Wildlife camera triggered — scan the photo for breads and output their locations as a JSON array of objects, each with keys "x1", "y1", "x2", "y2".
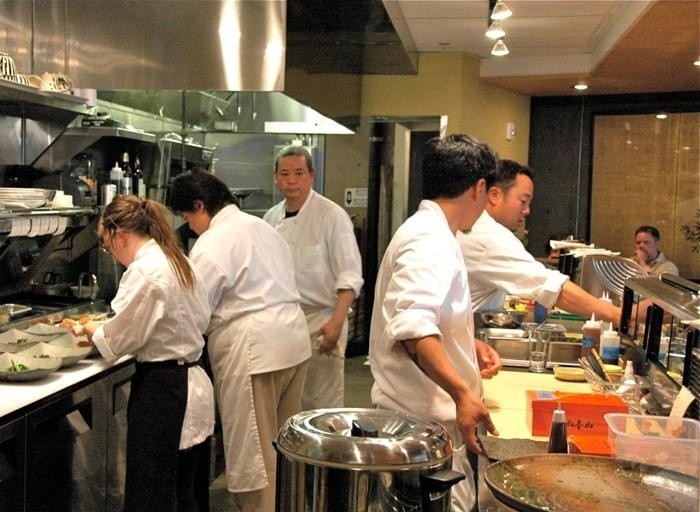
[
  {"x1": 61, "y1": 318, "x2": 76, "y2": 328},
  {"x1": 79, "y1": 313, "x2": 107, "y2": 324}
]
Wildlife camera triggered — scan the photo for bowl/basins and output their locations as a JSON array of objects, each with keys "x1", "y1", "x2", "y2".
[
  {"x1": 1, "y1": 303, "x2": 114, "y2": 381},
  {"x1": 0, "y1": 186, "x2": 55, "y2": 209},
  {"x1": 585, "y1": 372, "x2": 649, "y2": 399},
  {"x1": 70, "y1": 286, "x2": 99, "y2": 300},
  {"x1": 480, "y1": 309, "x2": 528, "y2": 328}
]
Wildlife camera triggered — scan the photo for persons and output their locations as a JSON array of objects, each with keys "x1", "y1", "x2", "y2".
[
  {"x1": 542, "y1": 233, "x2": 574, "y2": 260},
  {"x1": 366, "y1": 131, "x2": 505, "y2": 511},
  {"x1": 260, "y1": 146, "x2": 366, "y2": 410},
  {"x1": 69, "y1": 191, "x2": 217, "y2": 512},
  {"x1": 625, "y1": 225, "x2": 681, "y2": 279},
  {"x1": 450, "y1": 158, "x2": 674, "y2": 330},
  {"x1": 166, "y1": 165, "x2": 315, "y2": 512}
]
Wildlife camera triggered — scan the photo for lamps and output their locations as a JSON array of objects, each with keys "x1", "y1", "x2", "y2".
[{"x1": 483, "y1": 0, "x2": 513, "y2": 59}]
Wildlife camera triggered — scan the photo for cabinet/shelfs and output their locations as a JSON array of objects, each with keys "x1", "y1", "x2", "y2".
[{"x1": 0, "y1": 365, "x2": 136, "y2": 512}]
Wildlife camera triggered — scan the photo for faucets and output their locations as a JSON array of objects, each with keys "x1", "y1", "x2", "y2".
[{"x1": 76, "y1": 269, "x2": 99, "y2": 304}]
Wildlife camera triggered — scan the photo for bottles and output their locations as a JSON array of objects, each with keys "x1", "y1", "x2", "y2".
[
  {"x1": 657, "y1": 332, "x2": 670, "y2": 368},
  {"x1": 624, "y1": 360, "x2": 634, "y2": 376},
  {"x1": 547, "y1": 409, "x2": 569, "y2": 453},
  {"x1": 578, "y1": 311, "x2": 621, "y2": 366},
  {"x1": 82, "y1": 149, "x2": 147, "y2": 207},
  {"x1": 600, "y1": 289, "x2": 612, "y2": 304}
]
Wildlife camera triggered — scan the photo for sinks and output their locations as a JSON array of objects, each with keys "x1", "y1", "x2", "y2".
[
  {"x1": 483, "y1": 330, "x2": 595, "y2": 366},
  {"x1": 1, "y1": 291, "x2": 92, "y2": 309}
]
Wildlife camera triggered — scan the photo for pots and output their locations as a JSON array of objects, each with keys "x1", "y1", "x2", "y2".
[{"x1": 271, "y1": 406, "x2": 465, "y2": 511}]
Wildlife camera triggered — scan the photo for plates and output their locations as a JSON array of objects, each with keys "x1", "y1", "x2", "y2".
[
  {"x1": 227, "y1": 187, "x2": 262, "y2": 194},
  {"x1": 551, "y1": 366, "x2": 586, "y2": 381},
  {"x1": 484, "y1": 453, "x2": 699, "y2": 512}
]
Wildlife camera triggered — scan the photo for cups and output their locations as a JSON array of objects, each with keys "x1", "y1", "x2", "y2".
[
  {"x1": 527, "y1": 327, "x2": 551, "y2": 371},
  {"x1": 0, "y1": 52, "x2": 74, "y2": 95},
  {"x1": 53, "y1": 189, "x2": 74, "y2": 208},
  {"x1": 73, "y1": 87, "x2": 99, "y2": 108}
]
